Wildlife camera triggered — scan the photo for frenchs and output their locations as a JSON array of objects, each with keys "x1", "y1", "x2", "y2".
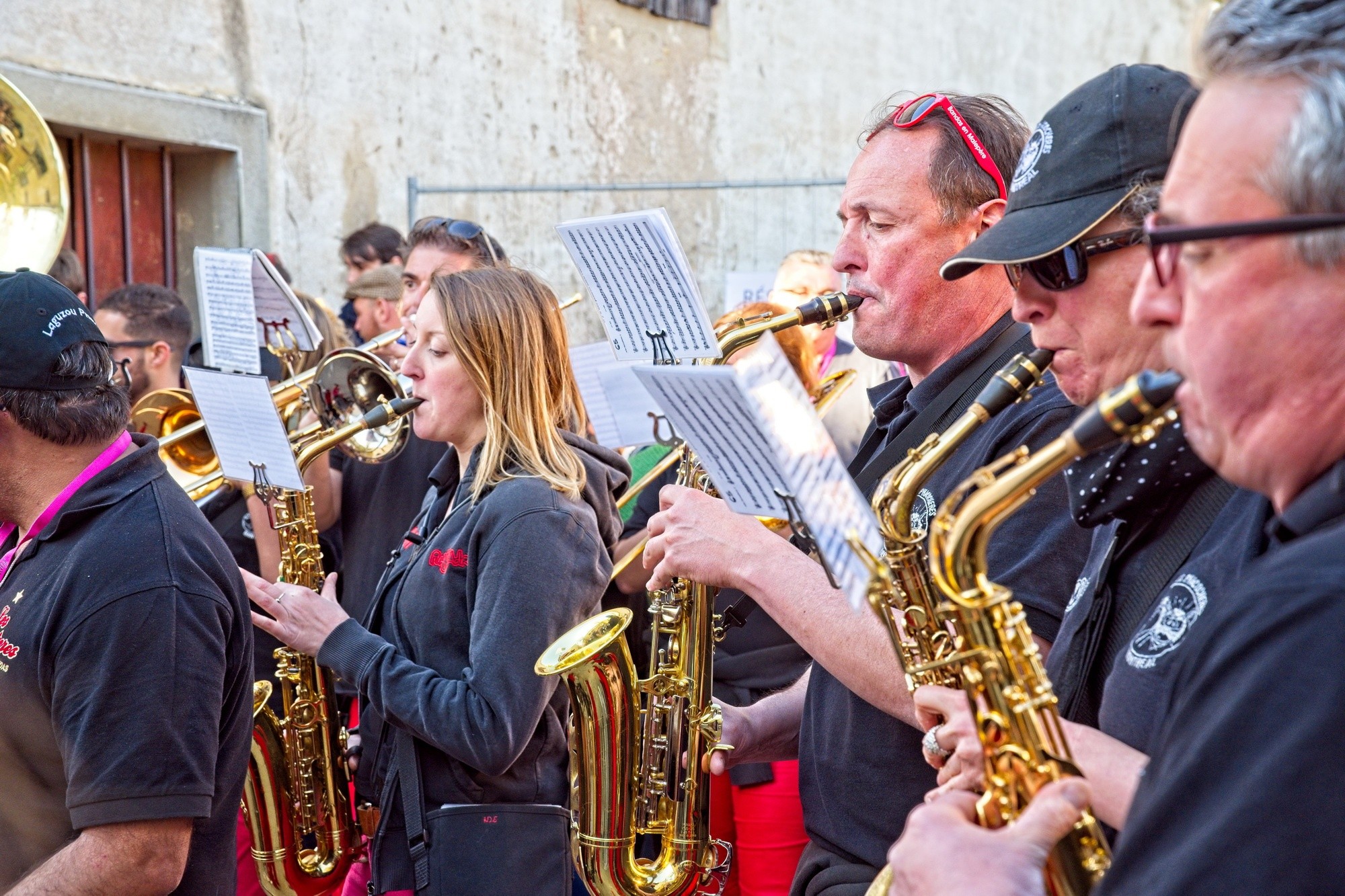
[{"x1": 0, "y1": 72, "x2": 73, "y2": 276}]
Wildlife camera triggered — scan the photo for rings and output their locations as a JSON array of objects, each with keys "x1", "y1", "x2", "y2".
[
  {"x1": 925, "y1": 725, "x2": 952, "y2": 759},
  {"x1": 279, "y1": 592, "x2": 287, "y2": 605}
]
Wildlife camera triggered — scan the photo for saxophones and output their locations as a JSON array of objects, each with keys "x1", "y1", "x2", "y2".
[
  {"x1": 830, "y1": 340, "x2": 1188, "y2": 896},
  {"x1": 237, "y1": 392, "x2": 424, "y2": 896},
  {"x1": 526, "y1": 291, "x2": 868, "y2": 896}
]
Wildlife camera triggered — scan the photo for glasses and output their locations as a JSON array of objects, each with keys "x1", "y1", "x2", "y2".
[
  {"x1": 866, "y1": 93, "x2": 1008, "y2": 202},
  {"x1": 1003, "y1": 227, "x2": 1144, "y2": 290},
  {"x1": 1143, "y1": 212, "x2": 1344, "y2": 288},
  {"x1": 412, "y1": 215, "x2": 500, "y2": 269}
]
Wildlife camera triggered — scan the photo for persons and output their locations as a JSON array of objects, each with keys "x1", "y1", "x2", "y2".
[
  {"x1": 0, "y1": 268, "x2": 257, "y2": 896},
  {"x1": 607, "y1": 2, "x2": 1344, "y2": 896},
  {"x1": 16, "y1": 215, "x2": 634, "y2": 896}
]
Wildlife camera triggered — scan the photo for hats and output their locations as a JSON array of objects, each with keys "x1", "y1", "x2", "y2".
[
  {"x1": 0, "y1": 267, "x2": 118, "y2": 391},
  {"x1": 345, "y1": 266, "x2": 405, "y2": 301},
  {"x1": 939, "y1": 63, "x2": 1203, "y2": 281}
]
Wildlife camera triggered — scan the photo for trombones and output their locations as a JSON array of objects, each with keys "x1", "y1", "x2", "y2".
[
  {"x1": 610, "y1": 367, "x2": 856, "y2": 583},
  {"x1": 126, "y1": 288, "x2": 585, "y2": 512}
]
